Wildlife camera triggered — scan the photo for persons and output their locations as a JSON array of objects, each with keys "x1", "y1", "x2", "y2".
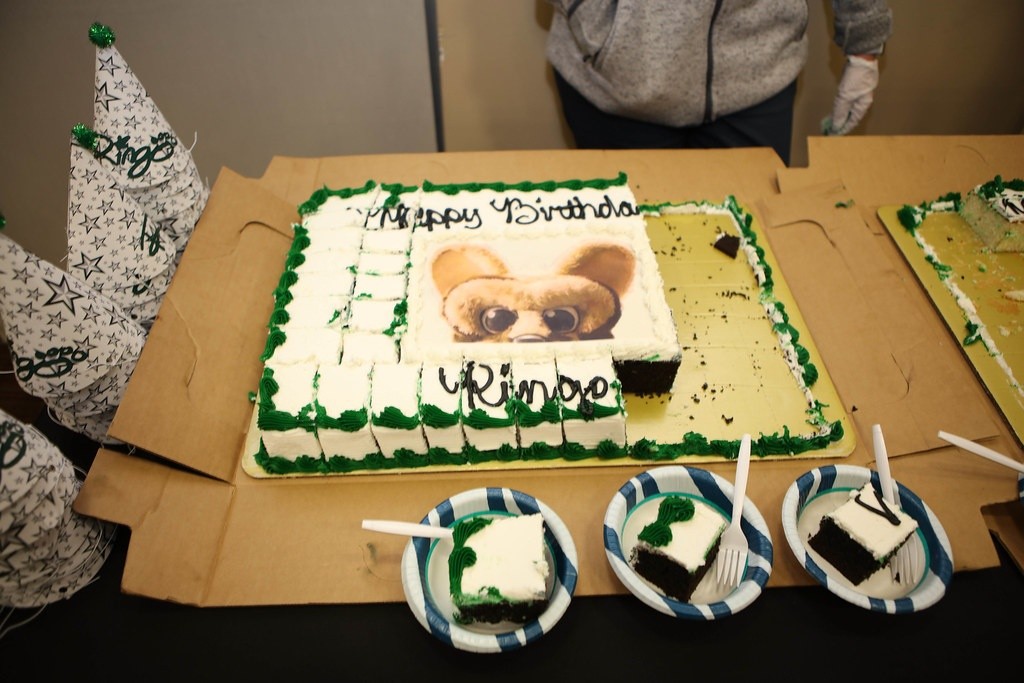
[{"x1": 544, "y1": 0, "x2": 892, "y2": 162}]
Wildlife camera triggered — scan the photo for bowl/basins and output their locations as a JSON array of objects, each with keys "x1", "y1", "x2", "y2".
[
  {"x1": 399, "y1": 484, "x2": 580, "y2": 655},
  {"x1": 780, "y1": 463, "x2": 954, "y2": 616},
  {"x1": 603, "y1": 464, "x2": 773, "y2": 623}
]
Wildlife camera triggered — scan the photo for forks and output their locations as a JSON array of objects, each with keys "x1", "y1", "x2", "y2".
[
  {"x1": 871, "y1": 422, "x2": 919, "y2": 587},
  {"x1": 716, "y1": 432, "x2": 751, "y2": 589}
]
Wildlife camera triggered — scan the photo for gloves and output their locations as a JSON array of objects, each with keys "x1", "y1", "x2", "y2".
[{"x1": 821, "y1": 55, "x2": 878, "y2": 137}]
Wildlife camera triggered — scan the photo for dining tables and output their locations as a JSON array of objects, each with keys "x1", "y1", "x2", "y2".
[{"x1": 0, "y1": 341, "x2": 1024, "y2": 683}]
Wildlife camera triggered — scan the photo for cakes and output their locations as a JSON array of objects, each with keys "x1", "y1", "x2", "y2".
[
  {"x1": 446, "y1": 512, "x2": 548, "y2": 625},
  {"x1": 895, "y1": 173, "x2": 1023, "y2": 395},
  {"x1": 252, "y1": 172, "x2": 842, "y2": 474},
  {"x1": 808, "y1": 483, "x2": 918, "y2": 587},
  {"x1": 627, "y1": 494, "x2": 726, "y2": 605}
]
